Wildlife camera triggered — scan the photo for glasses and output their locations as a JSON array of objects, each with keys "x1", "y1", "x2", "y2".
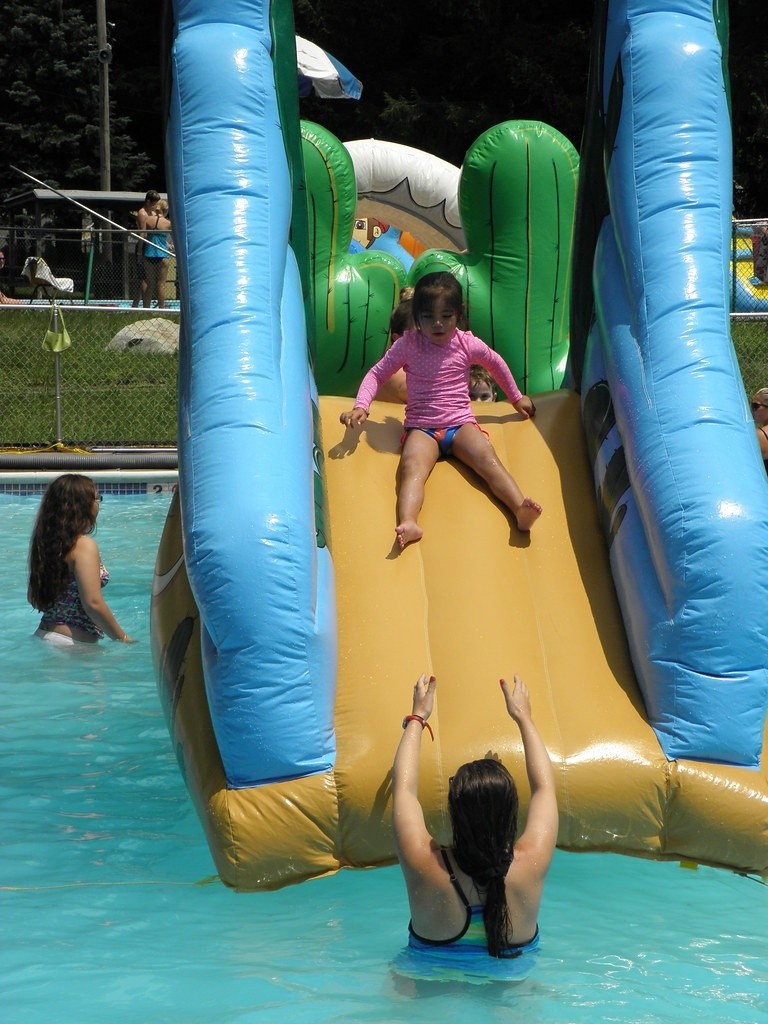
[
  {"x1": 750, "y1": 403, "x2": 768, "y2": 411},
  {"x1": 94, "y1": 494, "x2": 102, "y2": 505}
]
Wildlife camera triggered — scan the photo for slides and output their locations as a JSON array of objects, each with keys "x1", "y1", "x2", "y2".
[{"x1": 145, "y1": 0, "x2": 767, "y2": 894}]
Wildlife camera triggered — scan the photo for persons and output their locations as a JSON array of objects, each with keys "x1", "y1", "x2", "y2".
[
  {"x1": 339, "y1": 272, "x2": 543, "y2": 549},
  {"x1": 132, "y1": 191, "x2": 171, "y2": 309},
  {"x1": 752, "y1": 388, "x2": 768, "y2": 475},
  {"x1": 390, "y1": 671, "x2": 558, "y2": 955},
  {"x1": 27, "y1": 474, "x2": 132, "y2": 649},
  {"x1": 0, "y1": 251, "x2": 25, "y2": 305}
]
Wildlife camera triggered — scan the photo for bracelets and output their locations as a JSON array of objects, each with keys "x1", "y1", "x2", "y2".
[
  {"x1": 115, "y1": 635, "x2": 127, "y2": 642},
  {"x1": 413, "y1": 715, "x2": 424, "y2": 721}
]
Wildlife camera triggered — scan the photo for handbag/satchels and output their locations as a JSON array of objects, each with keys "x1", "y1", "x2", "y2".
[{"x1": 42, "y1": 303, "x2": 71, "y2": 352}]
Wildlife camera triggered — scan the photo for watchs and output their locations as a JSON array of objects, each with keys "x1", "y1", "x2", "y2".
[{"x1": 401, "y1": 716, "x2": 434, "y2": 741}]
